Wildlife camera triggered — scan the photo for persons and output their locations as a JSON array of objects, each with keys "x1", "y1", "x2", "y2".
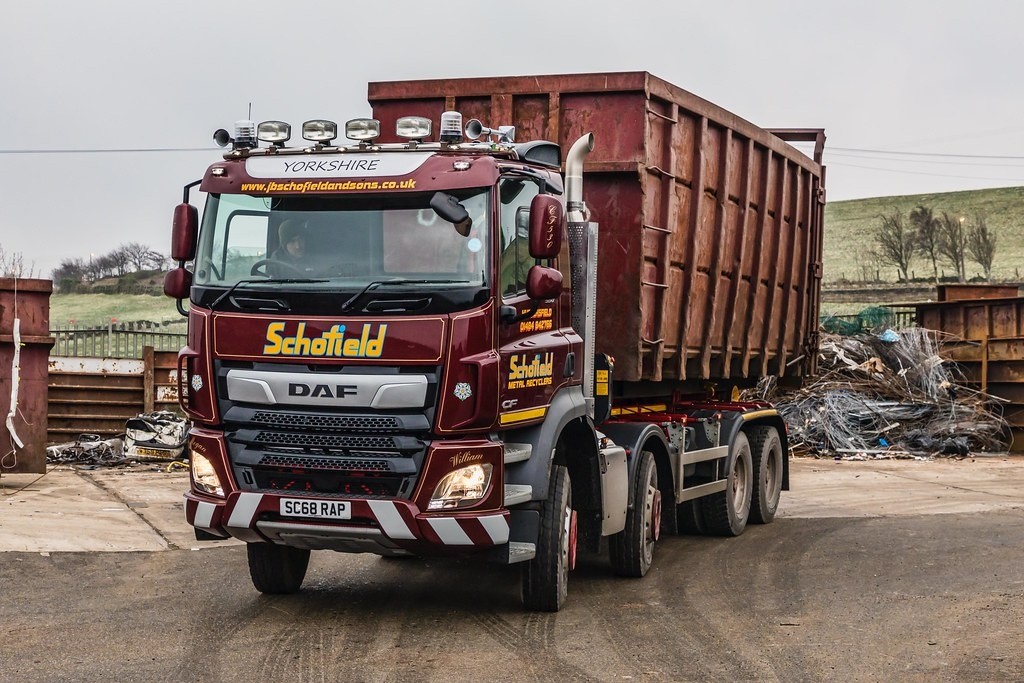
[{"x1": 265, "y1": 219, "x2": 324, "y2": 279}]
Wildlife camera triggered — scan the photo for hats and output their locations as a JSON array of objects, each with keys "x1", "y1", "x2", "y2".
[{"x1": 278, "y1": 219, "x2": 306, "y2": 248}]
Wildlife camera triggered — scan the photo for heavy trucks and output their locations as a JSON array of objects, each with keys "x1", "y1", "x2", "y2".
[{"x1": 164, "y1": 65, "x2": 828, "y2": 613}]
